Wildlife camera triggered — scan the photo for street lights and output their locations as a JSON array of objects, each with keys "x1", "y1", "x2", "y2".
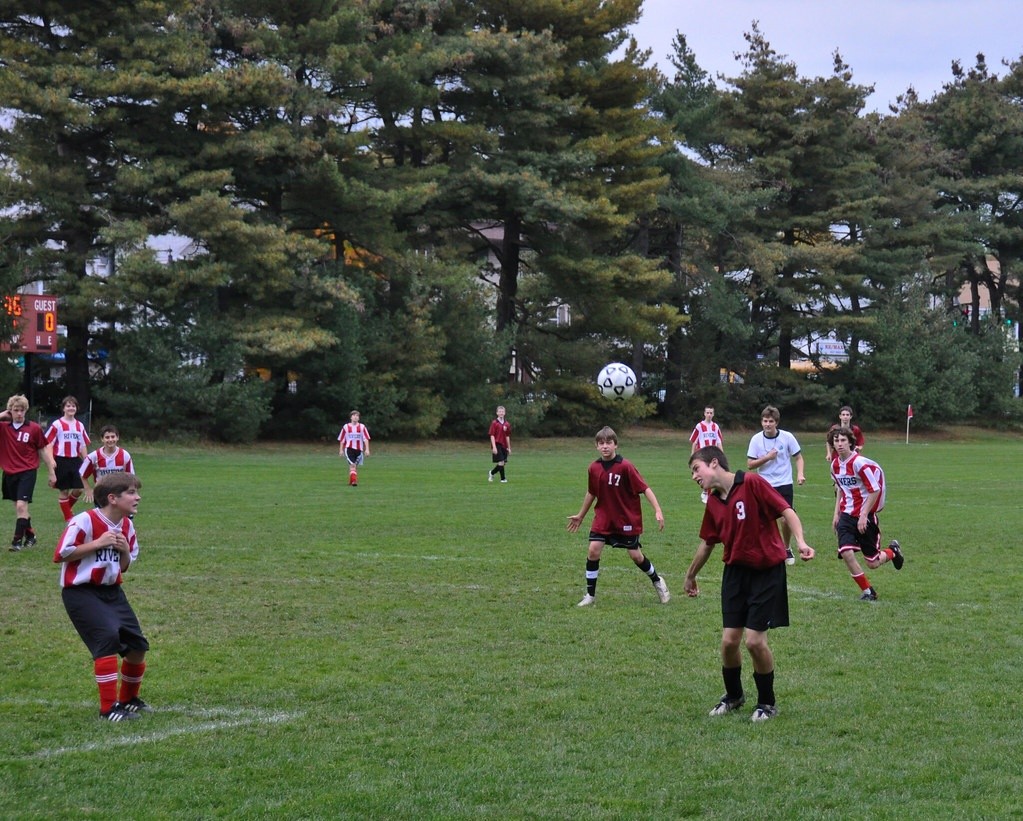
[{"x1": 117, "y1": 240, "x2": 177, "y2": 328}]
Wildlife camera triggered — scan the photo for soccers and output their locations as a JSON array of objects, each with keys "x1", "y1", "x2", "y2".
[{"x1": 597, "y1": 362, "x2": 636, "y2": 402}]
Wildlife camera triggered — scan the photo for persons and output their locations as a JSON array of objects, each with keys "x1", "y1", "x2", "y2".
[
  {"x1": 746, "y1": 405, "x2": 807, "y2": 566},
  {"x1": 827, "y1": 427, "x2": 904, "y2": 602},
  {"x1": 336, "y1": 410, "x2": 371, "y2": 487},
  {"x1": 51, "y1": 472, "x2": 158, "y2": 722},
  {"x1": 0, "y1": 394, "x2": 57, "y2": 552},
  {"x1": 825, "y1": 405, "x2": 865, "y2": 498},
  {"x1": 44, "y1": 395, "x2": 92, "y2": 522},
  {"x1": 566, "y1": 426, "x2": 671, "y2": 607},
  {"x1": 689, "y1": 402, "x2": 725, "y2": 504},
  {"x1": 488, "y1": 405, "x2": 512, "y2": 483},
  {"x1": 79, "y1": 424, "x2": 137, "y2": 525},
  {"x1": 683, "y1": 445, "x2": 815, "y2": 724}
]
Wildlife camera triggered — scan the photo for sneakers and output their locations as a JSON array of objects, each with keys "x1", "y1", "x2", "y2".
[
  {"x1": 653, "y1": 576, "x2": 671, "y2": 604},
  {"x1": 785, "y1": 547, "x2": 795, "y2": 566},
  {"x1": 99, "y1": 697, "x2": 153, "y2": 722},
  {"x1": 888, "y1": 540, "x2": 904, "y2": 570},
  {"x1": 701, "y1": 491, "x2": 708, "y2": 504},
  {"x1": 860, "y1": 586, "x2": 878, "y2": 601},
  {"x1": 709, "y1": 693, "x2": 746, "y2": 716},
  {"x1": 24, "y1": 535, "x2": 37, "y2": 548},
  {"x1": 500, "y1": 479, "x2": 507, "y2": 483},
  {"x1": 488, "y1": 470, "x2": 494, "y2": 482},
  {"x1": 578, "y1": 593, "x2": 595, "y2": 607},
  {"x1": 751, "y1": 703, "x2": 776, "y2": 722},
  {"x1": 9, "y1": 537, "x2": 22, "y2": 552}
]
